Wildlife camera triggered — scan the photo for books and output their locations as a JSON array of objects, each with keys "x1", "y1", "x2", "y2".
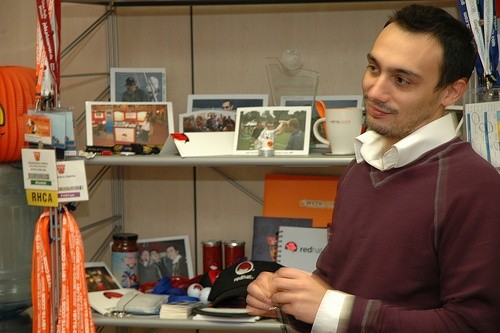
[{"x1": 251, "y1": 171, "x2": 342, "y2": 273}]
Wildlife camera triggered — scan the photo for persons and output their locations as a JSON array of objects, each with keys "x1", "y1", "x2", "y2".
[
  {"x1": 98, "y1": 77, "x2": 304, "y2": 152},
  {"x1": 243, "y1": 4, "x2": 500, "y2": 333},
  {"x1": 137, "y1": 246, "x2": 190, "y2": 284}
]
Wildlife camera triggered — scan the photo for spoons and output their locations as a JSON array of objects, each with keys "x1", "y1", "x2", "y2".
[{"x1": 316, "y1": 100, "x2": 327, "y2": 138}]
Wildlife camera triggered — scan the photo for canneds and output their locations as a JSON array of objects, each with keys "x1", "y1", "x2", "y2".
[{"x1": 111, "y1": 232, "x2": 140, "y2": 289}]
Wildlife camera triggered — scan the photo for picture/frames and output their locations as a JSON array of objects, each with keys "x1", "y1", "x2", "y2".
[
  {"x1": 187, "y1": 92, "x2": 269, "y2": 142},
  {"x1": 108, "y1": 234, "x2": 195, "y2": 285},
  {"x1": 85, "y1": 100, "x2": 175, "y2": 154},
  {"x1": 279, "y1": 94, "x2": 364, "y2": 149},
  {"x1": 109, "y1": 66, "x2": 168, "y2": 104},
  {"x1": 79, "y1": 262, "x2": 122, "y2": 292},
  {"x1": 233, "y1": 105, "x2": 313, "y2": 156}
]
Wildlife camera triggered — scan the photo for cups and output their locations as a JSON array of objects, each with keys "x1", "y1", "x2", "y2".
[
  {"x1": 313, "y1": 108, "x2": 362, "y2": 155},
  {"x1": 224, "y1": 240, "x2": 245, "y2": 269}
]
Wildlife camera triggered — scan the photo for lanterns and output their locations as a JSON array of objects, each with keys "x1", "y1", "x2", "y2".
[{"x1": 0, "y1": 65, "x2": 40, "y2": 164}]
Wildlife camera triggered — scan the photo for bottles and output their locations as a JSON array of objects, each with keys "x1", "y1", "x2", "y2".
[
  {"x1": 111, "y1": 233, "x2": 138, "y2": 288},
  {"x1": 202, "y1": 240, "x2": 223, "y2": 274}
]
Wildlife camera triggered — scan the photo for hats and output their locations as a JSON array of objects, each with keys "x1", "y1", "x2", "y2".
[
  {"x1": 124, "y1": 77, "x2": 135, "y2": 86},
  {"x1": 207, "y1": 260, "x2": 285, "y2": 308}
]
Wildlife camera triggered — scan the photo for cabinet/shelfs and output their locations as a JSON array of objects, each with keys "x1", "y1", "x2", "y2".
[{"x1": 40, "y1": 0, "x2": 427, "y2": 333}]
